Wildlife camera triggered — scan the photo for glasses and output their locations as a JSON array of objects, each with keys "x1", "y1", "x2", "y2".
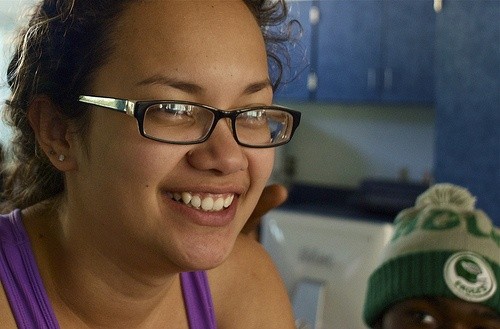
[{"x1": 72, "y1": 94, "x2": 300, "y2": 148}]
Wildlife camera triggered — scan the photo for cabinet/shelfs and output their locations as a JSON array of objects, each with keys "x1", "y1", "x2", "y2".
[
  {"x1": 312, "y1": 0, "x2": 436, "y2": 104},
  {"x1": 259, "y1": 0, "x2": 311, "y2": 107}
]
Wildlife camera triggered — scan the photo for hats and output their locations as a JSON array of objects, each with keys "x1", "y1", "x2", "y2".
[{"x1": 360, "y1": 182, "x2": 500, "y2": 329}]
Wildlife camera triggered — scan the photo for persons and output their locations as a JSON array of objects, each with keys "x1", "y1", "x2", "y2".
[
  {"x1": 362, "y1": 181, "x2": 500, "y2": 328},
  {"x1": 0, "y1": 2, "x2": 304, "y2": 329}
]
